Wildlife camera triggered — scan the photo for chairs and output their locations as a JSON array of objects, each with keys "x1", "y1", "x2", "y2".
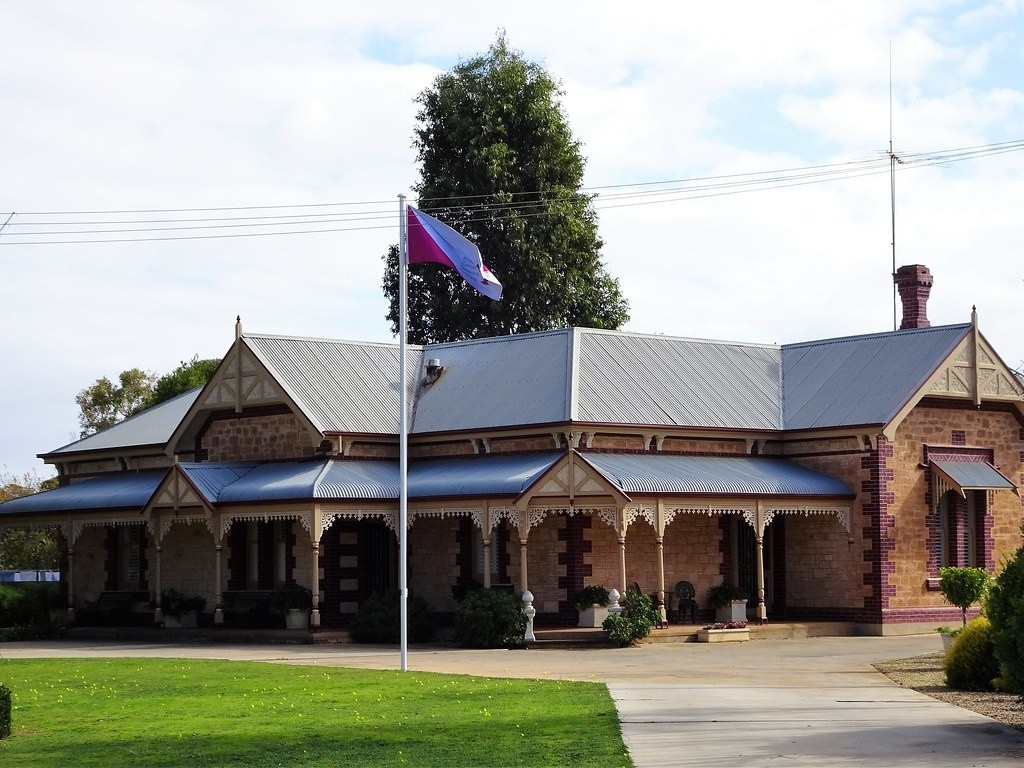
[
  {"x1": 634, "y1": 582, "x2": 655, "y2": 608},
  {"x1": 675, "y1": 581, "x2": 699, "y2": 625},
  {"x1": 655, "y1": 610, "x2": 668, "y2": 629}
]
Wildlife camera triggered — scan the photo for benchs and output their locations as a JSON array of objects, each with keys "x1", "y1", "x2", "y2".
[
  {"x1": 78, "y1": 591, "x2": 150, "y2": 626},
  {"x1": 215, "y1": 590, "x2": 276, "y2": 628}
]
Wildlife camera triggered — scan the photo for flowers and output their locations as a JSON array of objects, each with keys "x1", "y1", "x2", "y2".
[{"x1": 703, "y1": 620, "x2": 747, "y2": 630}]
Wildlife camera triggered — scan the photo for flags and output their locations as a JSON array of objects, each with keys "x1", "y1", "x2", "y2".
[{"x1": 405, "y1": 202, "x2": 504, "y2": 303}]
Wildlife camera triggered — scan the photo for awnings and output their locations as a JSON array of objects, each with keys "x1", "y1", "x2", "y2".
[{"x1": 931, "y1": 460, "x2": 1022, "y2": 498}]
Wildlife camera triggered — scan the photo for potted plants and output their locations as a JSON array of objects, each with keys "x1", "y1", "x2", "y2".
[
  {"x1": 269, "y1": 580, "x2": 313, "y2": 630},
  {"x1": 150, "y1": 587, "x2": 208, "y2": 628},
  {"x1": 570, "y1": 582, "x2": 610, "y2": 627},
  {"x1": 50, "y1": 595, "x2": 77, "y2": 625},
  {"x1": 933, "y1": 567, "x2": 992, "y2": 655},
  {"x1": 707, "y1": 579, "x2": 752, "y2": 622}
]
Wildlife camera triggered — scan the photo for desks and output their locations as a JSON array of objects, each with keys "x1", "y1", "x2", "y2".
[{"x1": 654, "y1": 592, "x2": 675, "y2": 623}]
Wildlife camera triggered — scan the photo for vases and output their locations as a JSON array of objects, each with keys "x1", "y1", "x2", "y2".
[{"x1": 696, "y1": 628, "x2": 750, "y2": 643}]
